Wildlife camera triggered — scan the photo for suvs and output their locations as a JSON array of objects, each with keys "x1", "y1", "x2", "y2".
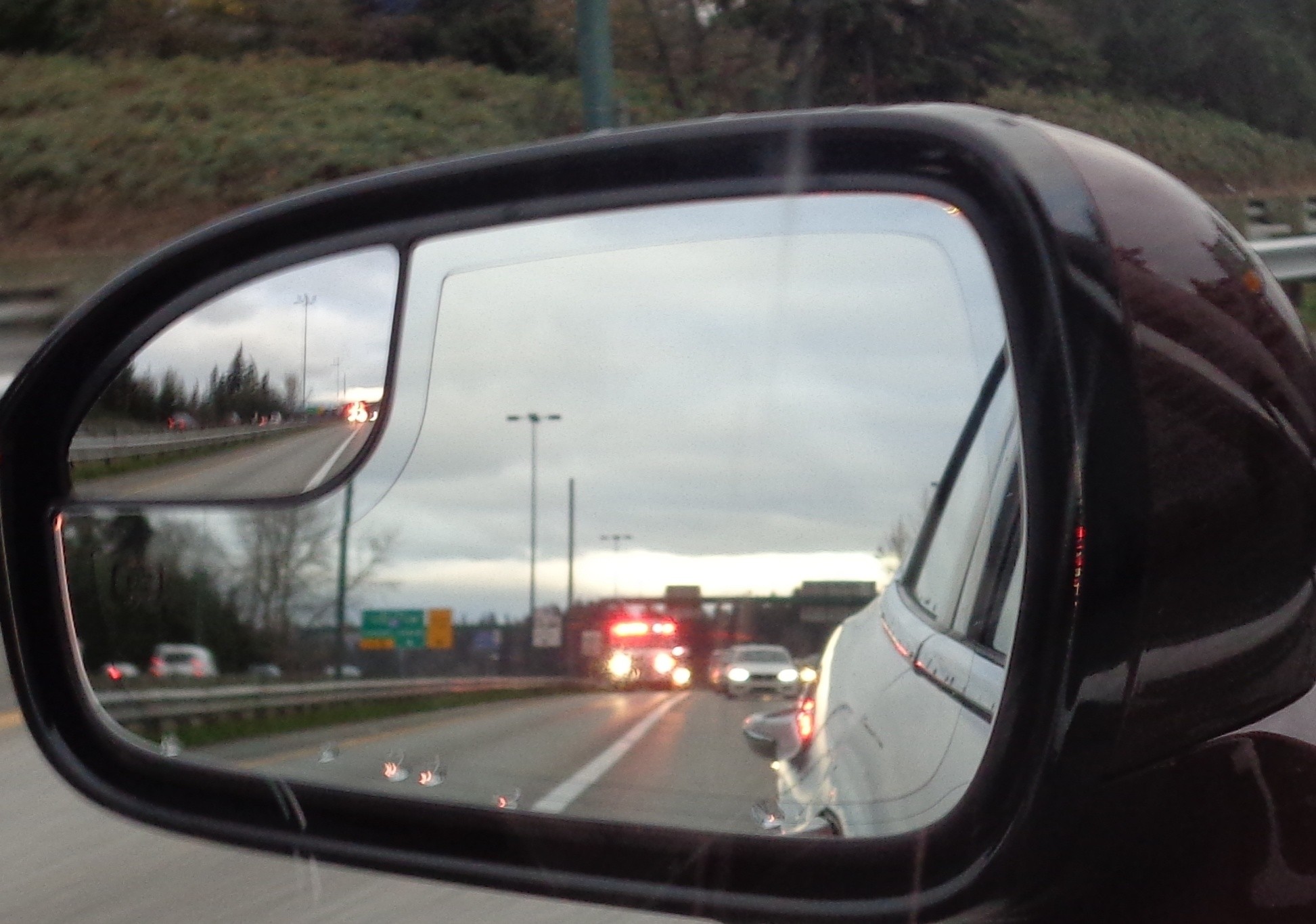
[{"x1": 602, "y1": 613, "x2": 694, "y2": 693}]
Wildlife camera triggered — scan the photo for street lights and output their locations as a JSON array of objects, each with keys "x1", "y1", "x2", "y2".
[
  {"x1": 506, "y1": 408, "x2": 564, "y2": 674},
  {"x1": 296, "y1": 292, "x2": 319, "y2": 422}
]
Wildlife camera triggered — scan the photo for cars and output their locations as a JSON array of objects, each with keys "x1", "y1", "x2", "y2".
[
  {"x1": 723, "y1": 644, "x2": 803, "y2": 700},
  {"x1": 151, "y1": 642, "x2": 219, "y2": 676}
]
